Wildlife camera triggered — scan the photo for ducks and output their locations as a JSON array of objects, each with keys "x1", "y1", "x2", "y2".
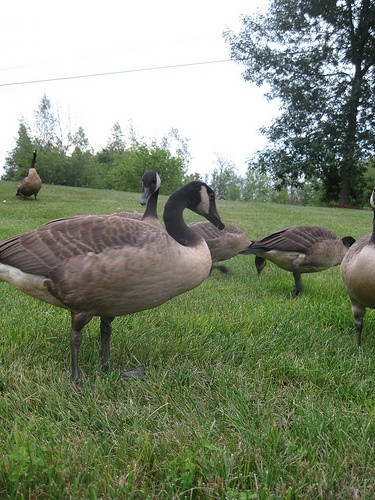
[
  {"x1": 16, "y1": 150, "x2": 42, "y2": 201},
  {"x1": 0, "y1": 169, "x2": 375, "y2": 385}
]
